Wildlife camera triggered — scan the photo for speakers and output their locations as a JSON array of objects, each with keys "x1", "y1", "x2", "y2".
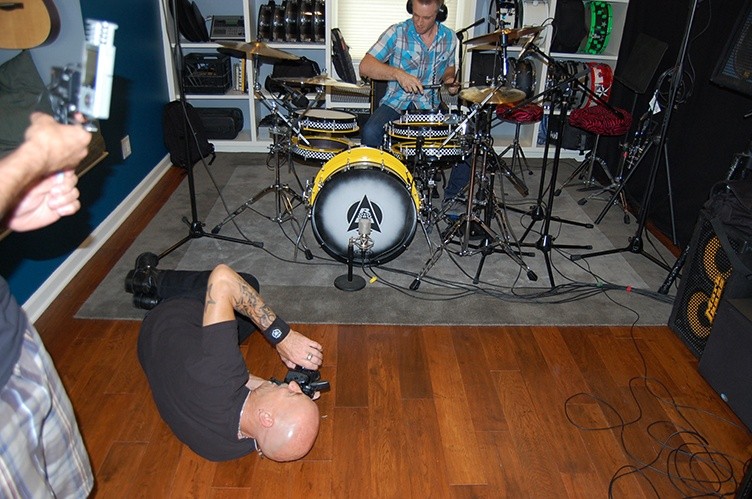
[
  {"x1": 667, "y1": 208, "x2": 752, "y2": 359},
  {"x1": 697, "y1": 298, "x2": 752, "y2": 436},
  {"x1": 709, "y1": 0, "x2": 752, "y2": 99}
]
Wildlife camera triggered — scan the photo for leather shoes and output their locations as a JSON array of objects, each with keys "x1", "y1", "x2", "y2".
[
  {"x1": 124, "y1": 251, "x2": 159, "y2": 295},
  {"x1": 133, "y1": 293, "x2": 161, "y2": 309}
]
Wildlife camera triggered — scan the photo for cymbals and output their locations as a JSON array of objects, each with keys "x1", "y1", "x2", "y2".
[
  {"x1": 459, "y1": 85, "x2": 527, "y2": 105},
  {"x1": 461, "y1": 22, "x2": 547, "y2": 54},
  {"x1": 213, "y1": 37, "x2": 303, "y2": 62},
  {"x1": 255, "y1": 0, "x2": 326, "y2": 45},
  {"x1": 270, "y1": 69, "x2": 372, "y2": 93}
]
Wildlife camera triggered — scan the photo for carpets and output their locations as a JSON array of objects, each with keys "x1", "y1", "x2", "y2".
[{"x1": 72, "y1": 152, "x2": 685, "y2": 327}]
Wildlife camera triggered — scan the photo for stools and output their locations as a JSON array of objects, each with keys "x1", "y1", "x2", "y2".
[
  {"x1": 494, "y1": 101, "x2": 544, "y2": 181},
  {"x1": 553, "y1": 104, "x2": 633, "y2": 206}
]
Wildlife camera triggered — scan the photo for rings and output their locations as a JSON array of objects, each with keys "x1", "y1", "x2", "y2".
[{"x1": 306, "y1": 352, "x2": 313, "y2": 360}]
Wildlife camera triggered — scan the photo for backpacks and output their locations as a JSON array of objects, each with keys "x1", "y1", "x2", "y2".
[{"x1": 162, "y1": 100, "x2": 215, "y2": 171}]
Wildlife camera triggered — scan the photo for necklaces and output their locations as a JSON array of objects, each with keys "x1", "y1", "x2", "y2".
[{"x1": 237, "y1": 389, "x2": 251, "y2": 440}]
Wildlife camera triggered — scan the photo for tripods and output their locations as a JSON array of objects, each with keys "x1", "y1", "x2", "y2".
[
  {"x1": 211, "y1": 104, "x2": 313, "y2": 261},
  {"x1": 157, "y1": 42, "x2": 265, "y2": 254},
  {"x1": 410, "y1": 79, "x2": 682, "y2": 294}
]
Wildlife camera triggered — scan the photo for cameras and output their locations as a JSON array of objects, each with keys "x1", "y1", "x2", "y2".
[{"x1": 284, "y1": 365, "x2": 330, "y2": 399}]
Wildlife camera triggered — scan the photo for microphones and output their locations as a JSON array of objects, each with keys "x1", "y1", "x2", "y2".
[
  {"x1": 358, "y1": 219, "x2": 371, "y2": 259},
  {"x1": 280, "y1": 80, "x2": 311, "y2": 107},
  {"x1": 406, "y1": 0, "x2": 448, "y2": 22}
]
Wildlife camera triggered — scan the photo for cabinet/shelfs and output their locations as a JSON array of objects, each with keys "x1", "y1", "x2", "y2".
[
  {"x1": 158, "y1": 0, "x2": 338, "y2": 153},
  {"x1": 454, "y1": 0, "x2": 630, "y2": 162}
]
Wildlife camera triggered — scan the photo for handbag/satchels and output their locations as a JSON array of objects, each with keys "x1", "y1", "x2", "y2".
[
  {"x1": 703, "y1": 176, "x2": 751, "y2": 247},
  {"x1": 331, "y1": 28, "x2": 357, "y2": 84},
  {"x1": 265, "y1": 56, "x2": 322, "y2": 94}
]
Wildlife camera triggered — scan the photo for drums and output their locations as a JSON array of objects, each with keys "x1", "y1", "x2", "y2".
[
  {"x1": 307, "y1": 145, "x2": 423, "y2": 268},
  {"x1": 486, "y1": 0, "x2": 616, "y2": 111},
  {"x1": 287, "y1": 106, "x2": 465, "y2": 170}
]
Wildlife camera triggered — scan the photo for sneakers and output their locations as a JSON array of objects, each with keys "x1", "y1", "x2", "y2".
[{"x1": 442, "y1": 200, "x2": 468, "y2": 215}]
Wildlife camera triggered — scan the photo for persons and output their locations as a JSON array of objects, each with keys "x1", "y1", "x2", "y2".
[
  {"x1": 0, "y1": 107, "x2": 98, "y2": 499},
  {"x1": 124, "y1": 251, "x2": 326, "y2": 464},
  {"x1": 359, "y1": 0, "x2": 480, "y2": 222}
]
